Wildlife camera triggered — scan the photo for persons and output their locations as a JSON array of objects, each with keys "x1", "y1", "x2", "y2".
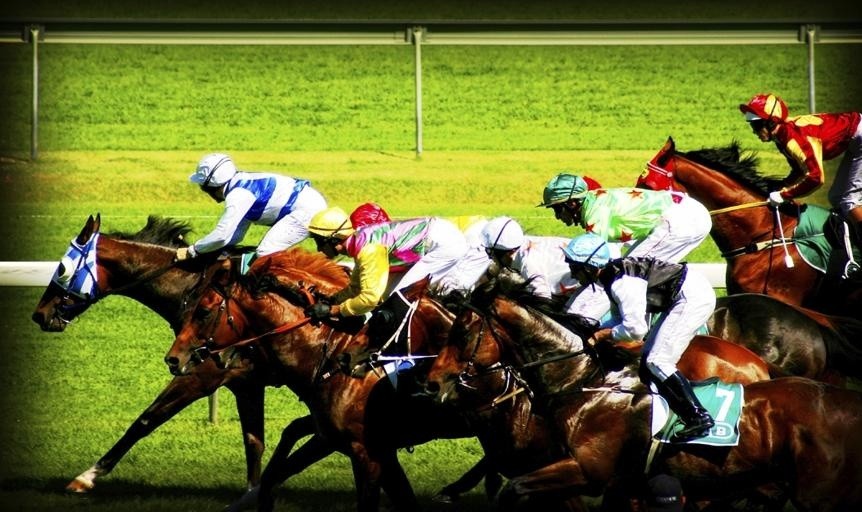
[
  {"x1": 741, "y1": 94, "x2": 862, "y2": 276},
  {"x1": 173, "y1": 153, "x2": 327, "y2": 263},
  {"x1": 642, "y1": 474, "x2": 688, "y2": 512},
  {"x1": 305, "y1": 174, "x2": 720, "y2": 444}
]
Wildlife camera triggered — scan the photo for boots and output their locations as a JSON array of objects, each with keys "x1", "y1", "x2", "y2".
[{"x1": 659, "y1": 368, "x2": 714, "y2": 443}]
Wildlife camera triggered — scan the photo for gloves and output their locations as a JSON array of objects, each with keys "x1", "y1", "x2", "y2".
[
  {"x1": 766, "y1": 190, "x2": 785, "y2": 209},
  {"x1": 172, "y1": 243, "x2": 197, "y2": 265},
  {"x1": 301, "y1": 301, "x2": 333, "y2": 323}
]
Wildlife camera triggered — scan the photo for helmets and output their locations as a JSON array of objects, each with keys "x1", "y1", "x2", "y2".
[
  {"x1": 308, "y1": 202, "x2": 391, "y2": 243},
  {"x1": 189, "y1": 152, "x2": 237, "y2": 187},
  {"x1": 558, "y1": 233, "x2": 612, "y2": 272},
  {"x1": 535, "y1": 172, "x2": 588, "y2": 210},
  {"x1": 480, "y1": 216, "x2": 525, "y2": 251},
  {"x1": 738, "y1": 92, "x2": 790, "y2": 125}
]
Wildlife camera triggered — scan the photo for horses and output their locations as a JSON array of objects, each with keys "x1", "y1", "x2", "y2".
[
  {"x1": 634, "y1": 136, "x2": 861, "y2": 352},
  {"x1": 31, "y1": 215, "x2": 862, "y2": 512}
]
